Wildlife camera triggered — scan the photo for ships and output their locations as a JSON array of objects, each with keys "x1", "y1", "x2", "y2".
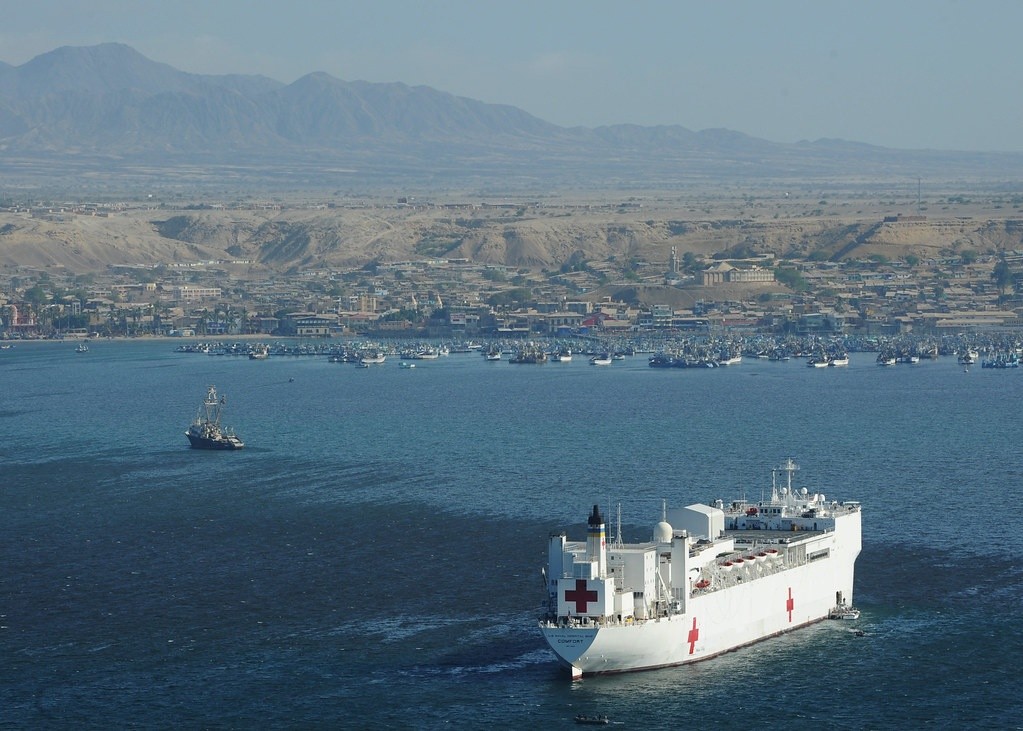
[{"x1": 537, "y1": 454, "x2": 862, "y2": 678}]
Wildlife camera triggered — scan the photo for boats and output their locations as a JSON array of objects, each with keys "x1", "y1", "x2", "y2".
[
  {"x1": 172, "y1": 337, "x2": 1023, "y2": 370},
  {"x1": 289, "y1": 376, "x2": 294, "y2": 383},
  {"x1": 185, "y1": 385, "x2": 244, "y2": 449},
  {"x1": 573, "y1": 716, "x2": 609, "y2": 725},
  {"x1": 963, "y1": 364, "x2": 969, "y2": 373},
  {"x1": 1, "y1": 344, "x2": 16, "y2": 349},
  {"x1": 75, "y1": 343, "x2": 89, "y2": 353}
]
